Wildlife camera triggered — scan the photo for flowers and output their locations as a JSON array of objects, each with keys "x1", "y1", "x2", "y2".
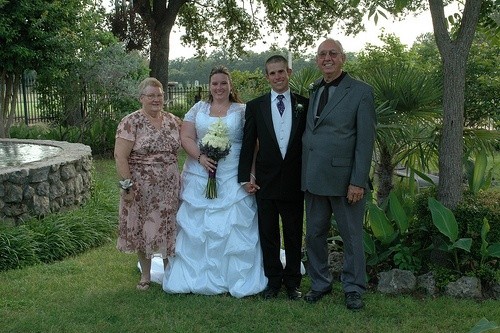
[
  {"x1": 294, "y1": 99, "x2": 304, "y2": 117},
  {"x1": 200, "y1": 117, "x2": 232, "y2": 199}
]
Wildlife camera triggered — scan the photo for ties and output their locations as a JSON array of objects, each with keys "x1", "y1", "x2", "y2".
[
  {"x1": 314, "y1": 83, "x2": 332, "y2": 127},
  {"x1": 276, "y1": 95, "x2": 285, "y2": 117}
]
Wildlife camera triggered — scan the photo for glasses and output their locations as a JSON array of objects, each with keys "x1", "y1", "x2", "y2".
[
  {"x1": 141, "y1": 94, "x2": 164, "y2": 101},
  {"x1": 318, "y1": 50, "x2": 342, "y2": 58}
]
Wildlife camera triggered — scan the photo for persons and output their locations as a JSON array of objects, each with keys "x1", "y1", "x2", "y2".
[
  {"x1": 115, "y1": 77, "x2": 183, "y2": 291},
  {"x1": 237, "y1": 55, "x2": 310, "y2": 300},
  {"x1": 162, "y1": 67, "x2": 268, "y2": 298},
  {"x1": 300, "y1": 38, "x2": 376, "y2": 308}
]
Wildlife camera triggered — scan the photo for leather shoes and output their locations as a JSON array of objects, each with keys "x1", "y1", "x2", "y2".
[
  {"x1": 264, "y1": 286, "x2": 282, "y2": 300},
  {"x1": 286, "y1": 285, "x2": 302, "y2": 300}
]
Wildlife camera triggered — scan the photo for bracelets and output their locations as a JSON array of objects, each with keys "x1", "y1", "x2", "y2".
[{"x1": 198, "y1": 152, "x2": 205, "y2": 163}]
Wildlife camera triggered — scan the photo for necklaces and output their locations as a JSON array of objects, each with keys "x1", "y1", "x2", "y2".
[{"x1": 219, "y1": 111, "x2": 220, "y2": 113}]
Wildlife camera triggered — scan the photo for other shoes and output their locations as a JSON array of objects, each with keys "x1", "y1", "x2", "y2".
[
  {"x1": 344, "y1": 290, "x2": 364, "y2": 311},
  {"x1": 136, "y1": 282, "x2": 150, "y2": 290},
  {"x1": 304, "y1": 287, "x2": 332, "y2": 302}
]
